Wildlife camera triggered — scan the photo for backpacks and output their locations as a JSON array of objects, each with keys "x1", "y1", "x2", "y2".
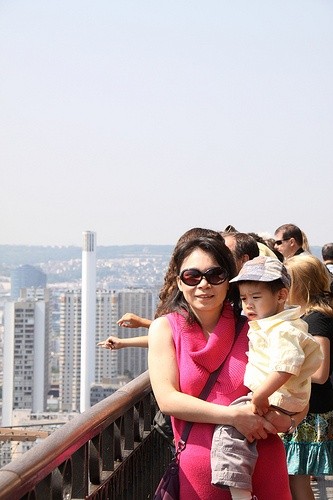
[{"x1": 153, "y1": 409, "x2": 176, "y2": 449}]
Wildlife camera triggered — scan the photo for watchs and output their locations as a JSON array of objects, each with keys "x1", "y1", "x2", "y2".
[{"x1": 288, "y1": 415, "x2": 296, "y2": 434}]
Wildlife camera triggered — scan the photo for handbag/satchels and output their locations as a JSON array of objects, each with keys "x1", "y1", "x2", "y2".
[{"x1": 152, "y1": 456, "x2": 180, "y2": 500}]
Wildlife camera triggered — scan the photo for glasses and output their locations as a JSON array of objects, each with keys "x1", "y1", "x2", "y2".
[
  {"x1": 275, "y1": 240, "x2": 282, "y2": 245},
  {"x1": 178, "y1": 266, "x2": 228, "y2": 287}
]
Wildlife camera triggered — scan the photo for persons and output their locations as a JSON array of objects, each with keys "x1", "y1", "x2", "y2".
[
  {"x1": 322, "y1": 242, "x2": 333, "y2": 273},
  {"x1": 248, "y1": 230, "x2": 309, "y2": 262},
  {"x1": 97, "y1": 231, "x2": 260, "y2": 349},
  {"x1": 221, "y1": 232, "x2": 259, "y2": 274},
  {"x1": 273, "y1": 222, "x2": 307, "y2": 298},
  {"x1": 210, "y1": 255, "x2": 322, "y2": 500},
  {"x1": 277, "y1": 259, "x2": 333, "y2": 500},
  {"x1": 148, "y1": 227, "x2": 309, "y2": 500}
]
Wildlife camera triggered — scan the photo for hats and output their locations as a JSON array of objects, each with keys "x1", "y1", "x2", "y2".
[{"x1": 228, "y1": 255, "x2": 292, "y2": 293}]
